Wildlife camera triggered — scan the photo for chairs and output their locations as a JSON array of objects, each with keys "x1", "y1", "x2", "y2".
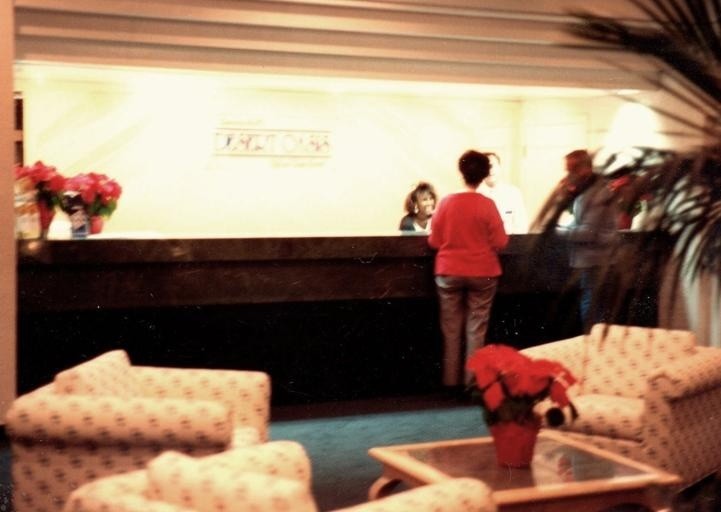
[
  {"x1": 519, "y1": 323, "x2": 721, "y2": 497},
  {"x1": 62, "y1": 440, "x2": 498, "y2": 512},
  {"x1": 4, "y1": 348, "x2": 271, "y2": 512}
]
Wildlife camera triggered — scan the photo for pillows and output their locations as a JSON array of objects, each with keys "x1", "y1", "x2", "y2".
[
  {"x1": 146, "y1": 451, "x2": 316, "y2": 512},
  {"x1": 54, "y1": 348, "x2": 133, "y2": 395},
  {"x1": 582, "y1": 325, "x2": 696, "y2": 401}
]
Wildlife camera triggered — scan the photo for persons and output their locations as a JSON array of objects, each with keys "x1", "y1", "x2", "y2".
[
  {"x1": 427, "y1": 149, "x2": 509, "y2": 397},
  {"x1": 477, "y1": 152, "x2": 531, "y2": 235},
  {"x1": 399, "y1": 181, "x2": 437, "y2": 232},
  {"x1": 554, "y1": 149, "x2": 618, "y2": 335}
]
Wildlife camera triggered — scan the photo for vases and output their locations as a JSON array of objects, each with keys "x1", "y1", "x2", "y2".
[
  {"x1": 88, "y1": 216, "x2": 103, "y2": 236},
  {"x1": 487, "y1": 414, "x2": 541, "y2": 467},
  {"x1": 35, "y1": 200, "x2": 56, "y2": 238}
]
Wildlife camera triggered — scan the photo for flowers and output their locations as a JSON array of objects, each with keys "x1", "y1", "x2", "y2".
[
  {"x1": 15, "y1": 160, "x2": 65, "y2": 207},
  {"x1": 59, "y1": 172, "x2": 122, "y2": 220},
  {"x1": 462, "y1": 344, "x2": 578, "y2": 424}
]
